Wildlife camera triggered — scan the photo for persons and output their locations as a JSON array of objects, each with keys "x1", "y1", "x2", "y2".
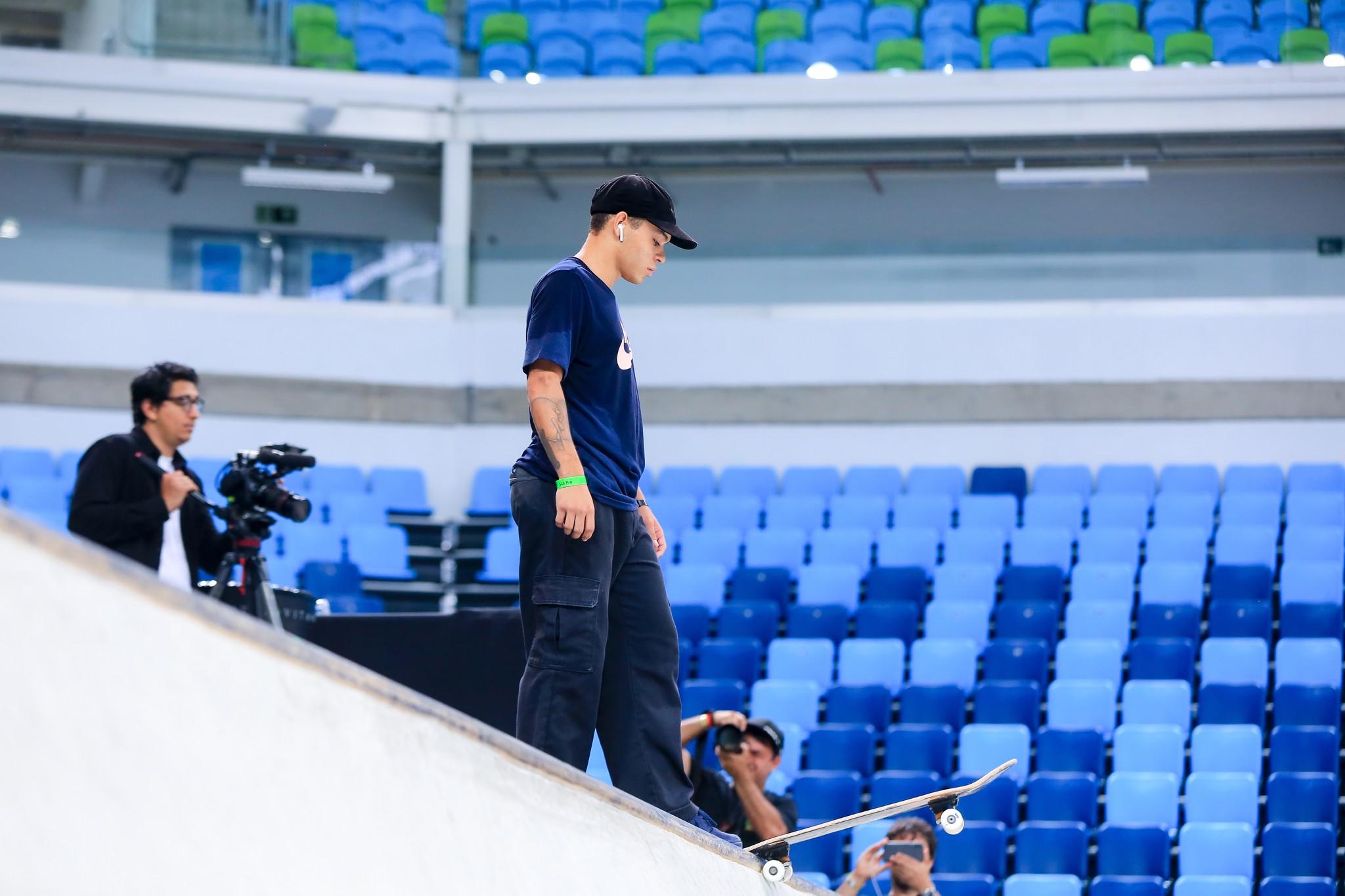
[
  {"x1": 680, "y1": 708, "x2": 798, "y2": 862},
  {"x1": 833, "y1": 816, "x2": 943, "y2": 896},
  {"x1": 68, "y1": 360, "x2": 236, "y2": 593},
  {"x1": 509, "y1": 172, "x2": 743, "y2": 846}
]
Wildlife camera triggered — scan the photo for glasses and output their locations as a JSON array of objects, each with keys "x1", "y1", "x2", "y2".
[{"x1": 152, "y1": 394, "x2": 204, "y2": 406}]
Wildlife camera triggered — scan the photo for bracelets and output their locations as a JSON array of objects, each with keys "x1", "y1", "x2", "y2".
[
  {"x1": 921, "y1": 887, "x2": 938, "y2": 896},
  {"x1": 636, "y1": 499, "x2": 648, "y2": 508},
  {"x1": 555, "y1": 475, "x2": 588, "y2": 490},
  {"x1": 699, "y1": 711, "x2": 714, "y2": 732}
]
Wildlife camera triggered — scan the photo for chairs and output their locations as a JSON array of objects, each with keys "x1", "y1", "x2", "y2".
[
  {"x1": 0, "y1": 445, "x2": 1345, "y2": 896},
  {"x1": 247, "y1": 0, "x2": 1345, "y2": 81}
]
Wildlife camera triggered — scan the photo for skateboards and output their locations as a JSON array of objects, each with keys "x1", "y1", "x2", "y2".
[{"x1": 743, "y1": 758, "x2": 1018, "y2": 882}]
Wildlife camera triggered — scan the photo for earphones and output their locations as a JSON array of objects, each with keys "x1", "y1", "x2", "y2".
[{"x1": 618, "y1": 223, "x2": 623, "y2": 242}]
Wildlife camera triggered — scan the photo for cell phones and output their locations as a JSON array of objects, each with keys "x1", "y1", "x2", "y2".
[{"x1": 883, "y1": 842, "x2": 924, "y2": 863}]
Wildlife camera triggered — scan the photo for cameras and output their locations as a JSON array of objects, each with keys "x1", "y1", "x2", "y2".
[{"x1": 713, "y1": 723, "x2": 745, "y2": 756}]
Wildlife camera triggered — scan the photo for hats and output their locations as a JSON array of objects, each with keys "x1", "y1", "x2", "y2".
[
  {"x1": 745, "y1": 718, "x2": 783, "y2": 756},
  {"x1": 589, "y1": 173, "x2": 698, "y2": 250}
]
